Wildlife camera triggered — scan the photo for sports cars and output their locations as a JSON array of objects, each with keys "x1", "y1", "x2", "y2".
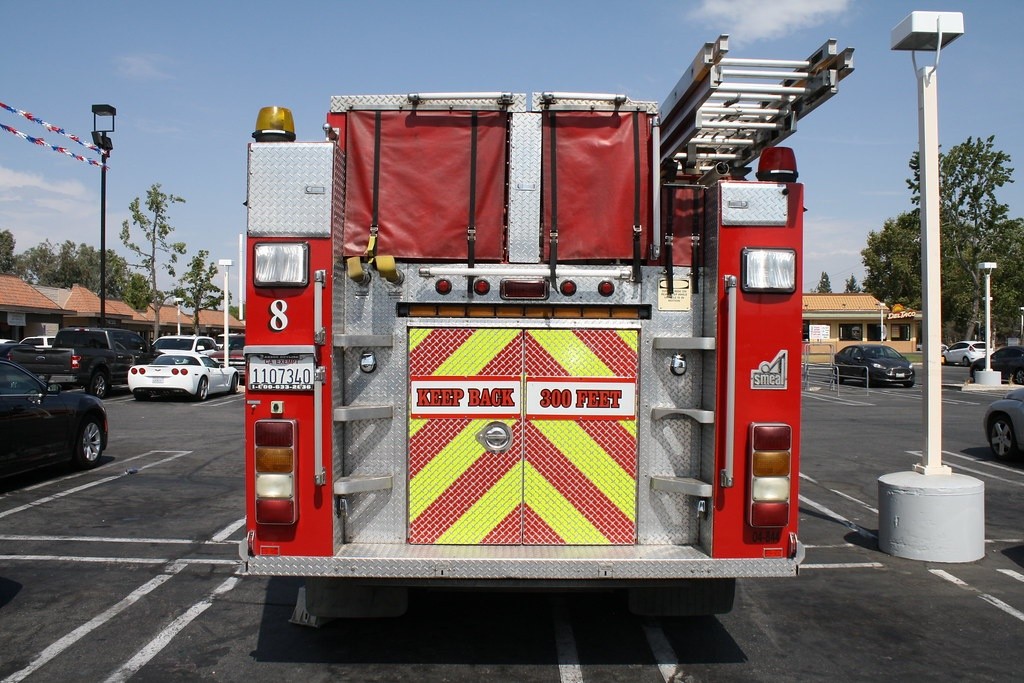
[{"x1": 127, "y1": 351, "x2": 240, "y2": 402}]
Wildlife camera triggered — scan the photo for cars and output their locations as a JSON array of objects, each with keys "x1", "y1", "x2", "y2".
[
  {"x1": 208, "y1": 334, "x2": 247, "y2": 384},
  {"x1": 916, "y1": 342, "x2": 948, "y2": 351},
  {"x1": 17, "y1": 335, "x2": 57, "y2": 351},
  {"x1": 834, "y1": 344, "x2": 916, "y2": 388},
  {"x1": 151, "y1": 334, "x2": 226, "y2": 357},
  {"x1": 942, "y1": 340, "x2": 994, "y2": 366},
  {"x1": 969, "y1": 346, "x2": 1024, "y2": 385},
  {"x1": 0, "y1": 358, "x2": 109, "y2": 481},
  {"x1": 983, "y1": 386, "x2": 1024, "y2": 462},
  {"x1": 0, "y1": 338, "x2": 17, "y2": 345}
]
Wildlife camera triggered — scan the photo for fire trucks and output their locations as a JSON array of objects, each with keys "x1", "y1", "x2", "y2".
[{"x1": 236, "y1": 32, "x2": 856, "y2": 633}]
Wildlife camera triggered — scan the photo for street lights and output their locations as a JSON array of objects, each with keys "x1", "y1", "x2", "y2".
[
  {"x1": 219, "y1": 259, "x2": 236, "y2": 367},
  {"x1": 880, "y1": 302, "x2": 885, "y2": 346},
  {"x1": 977, "y1": 261, "x2": 999, "y2": 371},
  {"x1": 889, "y1": 9, "x2": 965, "y2": 475},
  {"x1": 174, "y1": 297, "x2": 185, "y2": 336},
  {"x1": 91, "y1": 103, "x2": 119, "y2": 326}
]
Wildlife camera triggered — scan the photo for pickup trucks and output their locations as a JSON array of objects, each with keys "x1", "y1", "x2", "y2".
[{"x1": 0, "y1": 326, "x2": 152, "y2": 399}]
